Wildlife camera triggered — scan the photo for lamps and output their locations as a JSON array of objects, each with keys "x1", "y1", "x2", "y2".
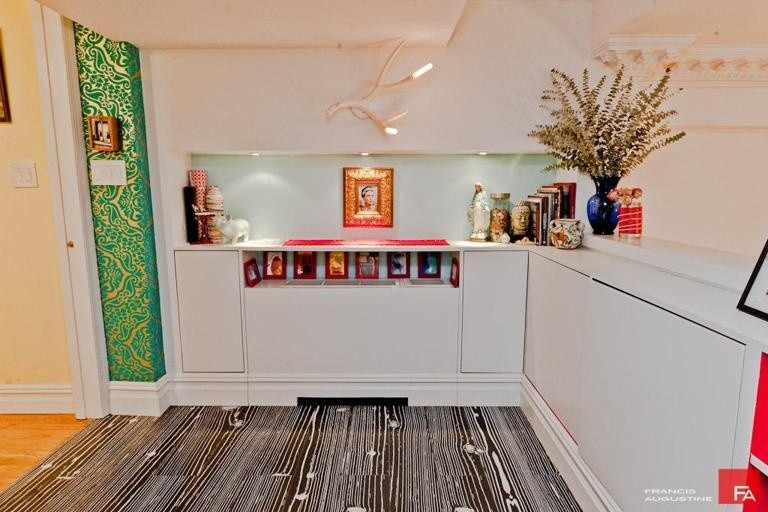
[{"x1": 324, "y1": 39, "x2": 434, "y2": 137}]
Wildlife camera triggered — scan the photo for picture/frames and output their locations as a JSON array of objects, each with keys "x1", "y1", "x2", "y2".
[
  {"x1": 86, "y1": 114, "x2": 121, "y2": 153},
  {"x1": 736, "y1": 235, "x2": 768, "y2": 324},
  {"x1": 342, "y1": 165, "x2": 395, "y2": 229},
  {"x1": 243, "y1": 251, "x2": 460, "y2": 287}
]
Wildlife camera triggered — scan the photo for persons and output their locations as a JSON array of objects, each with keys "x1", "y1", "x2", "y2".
[
  {"x1": 510, "y1": 200, "x2": 530, "y2": 235},
  {"x1": 359, "y1": 185, "x2": 377, "y2": 211},
  {"x1": 467, "y1": 182, "x2": 490, "y2": 234}
]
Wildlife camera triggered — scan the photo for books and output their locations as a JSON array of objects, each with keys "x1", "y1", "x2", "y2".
[{"x1": 525, "y1": 182, "x2": 576, "y2": 246}]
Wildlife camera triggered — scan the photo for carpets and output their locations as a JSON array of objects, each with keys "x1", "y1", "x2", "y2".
[{"x1": 0, "y1": 404, "x2": 586, "y2": 512}]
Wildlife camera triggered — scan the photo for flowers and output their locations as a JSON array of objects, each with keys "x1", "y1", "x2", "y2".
[{"x1": 610, "y1": 184, "x2": 648, "y2": 207}]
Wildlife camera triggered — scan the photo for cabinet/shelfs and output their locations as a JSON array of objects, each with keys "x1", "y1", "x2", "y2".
[
  {"x1": 172, "y1": 246, "x2": 244, "y2": 407},
  {"x1": 462, "y1": 248, "x2": 529, "y2": 407},
  {"x1": 518, "y1": 243, "x2": 746, "y2": 512},
  {"x1": 238, "y1": 246, "x2": 463, "y2": 407}
]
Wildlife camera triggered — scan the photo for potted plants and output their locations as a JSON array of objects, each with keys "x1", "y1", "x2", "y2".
[{"x1": 526, "y1": 59, "x2": 688, "y2": 236}]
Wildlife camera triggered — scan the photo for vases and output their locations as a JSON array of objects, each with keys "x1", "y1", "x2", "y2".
[
  {"x1": 187, "y1": 168, "x2": 207, "y2": 242},
  {"x1": 618, "y1": 206, "x2": 642, "y2": 239},
  {"x1": 205, "y1": 184, "x2": 224, "y2": 244},
  {"x1": 549, "y1": 218, "x2": 585, "y2": 249}
]
